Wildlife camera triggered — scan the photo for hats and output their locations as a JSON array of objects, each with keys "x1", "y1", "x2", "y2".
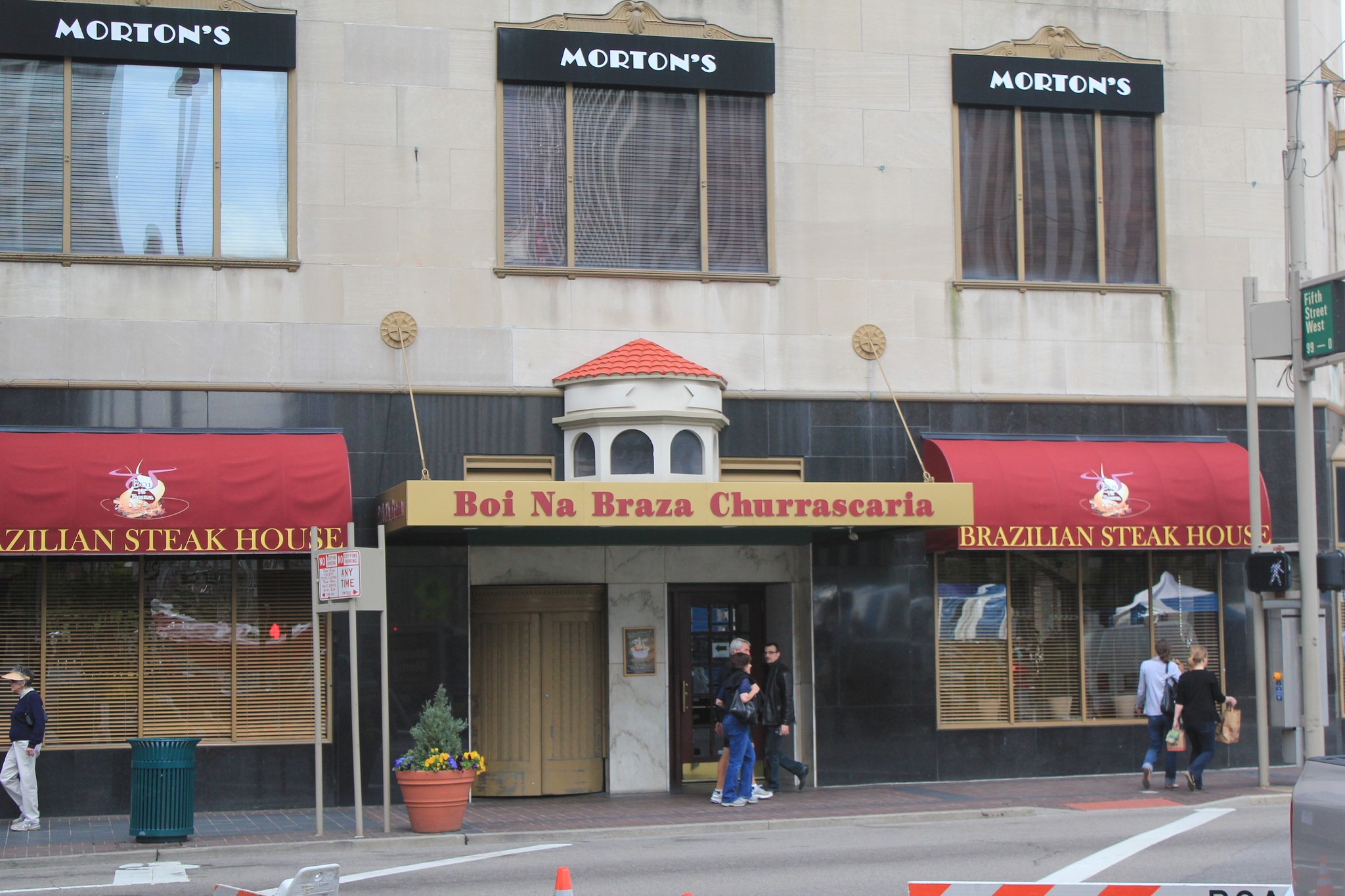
[{"x1": 1, "y1": 671, "x2": 30, "y2": 680}]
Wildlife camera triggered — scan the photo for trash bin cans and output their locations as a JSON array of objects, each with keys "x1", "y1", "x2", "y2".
[{"x1": 126, "y1": 736, "x2": 203, "y2": 845}]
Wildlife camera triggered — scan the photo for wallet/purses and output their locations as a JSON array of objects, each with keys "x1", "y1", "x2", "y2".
[{"x1": 1165, "y1": 729, "x2": 1179, "y2": 743}]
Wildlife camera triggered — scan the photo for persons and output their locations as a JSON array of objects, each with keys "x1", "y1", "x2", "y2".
[
  {"x1": 1135, "y1": 639, "x2": 1182, "y2": 789},
  {"x1": 1, "y1": 665, "x2": 45, "y2": 831},
  {"x1": 715, "y1": 653, "x2": 761, "y2": 807},
  {"x1": 756, "y1": 642, "x2": 811, "y2": 793},
  {"x1": 709, "y1": 638, "x2": 775, "y2": 803},
  {"x1": 1170, "y1": 647, "x2": 1239, "y2": 790}
]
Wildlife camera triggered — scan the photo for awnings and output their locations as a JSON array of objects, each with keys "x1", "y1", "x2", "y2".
[
  {"x1": 0, "y1": 426, "x2": 351, "y2": 557},
  {"x1": 918, "y1": 431, "x2": 1274, "y2": 554}
]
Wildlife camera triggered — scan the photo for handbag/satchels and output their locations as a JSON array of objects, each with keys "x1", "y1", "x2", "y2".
[
  {"x1": 25, "y1": 693, "x2": 48, "y2": 725},
  {"x1": 1215, "y1": 703, "x2": 1241, "y2": 744},
  {"x1": 728, "y1": 689, "x2": 757, "y2": 726},
  {"x1": 1160, "y1": 663, "x2": 1178, "y2": 714},
  {"x1": 1167, "y1": 727, "x2": 1186, "y2": 751}
]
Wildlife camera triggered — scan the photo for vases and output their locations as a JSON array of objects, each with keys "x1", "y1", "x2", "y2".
[{"x1": 395, "y1": 769, "x2": 470, "y2": 834}]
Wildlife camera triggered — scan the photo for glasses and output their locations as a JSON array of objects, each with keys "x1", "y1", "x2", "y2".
[
  {"x1": 10, "y1": 680, "x2": 23, "y2": 683},
  {"x1": 763, "y1": 651, "x2": 777, "y2": 654}
]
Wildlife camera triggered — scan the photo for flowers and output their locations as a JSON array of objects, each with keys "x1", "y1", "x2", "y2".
[{"x1": 390, "y1": 681, "x2": 486, "y2": 776}]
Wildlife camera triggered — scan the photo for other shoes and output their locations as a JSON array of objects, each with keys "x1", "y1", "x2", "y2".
[
  {"x1": 796, "y1": 764, "x2": 808, "y2": 790},
  {"x1": 1141, "y1": 767, "x2": 1151, "y2": 790},
  {"x1": 1165, "y1": 784, "x2": 1180, "y2": 788},
  {"x1": 1184, "y1": 770, "x2": 1196, "y2": 791},
  {"x1": 759, "y1": 786, "x2": 781, "y2": 794}
]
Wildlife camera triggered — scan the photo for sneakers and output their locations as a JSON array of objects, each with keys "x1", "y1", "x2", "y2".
[
  {"x1": 720, "y1": 798, "x2": 746, "y2": 806},
  {"x1": 10, "y1": 811, "x2": 40, "y2": 830},
  {"x1": 751, "y1": 784, "x2": 773, "y2": 798},
  {"x1": 710, "y1": 790, "x2": 722, "y2": 803},
  {"x1": 739, "y1": 796, "x2": 758, "y2": 803}
]
